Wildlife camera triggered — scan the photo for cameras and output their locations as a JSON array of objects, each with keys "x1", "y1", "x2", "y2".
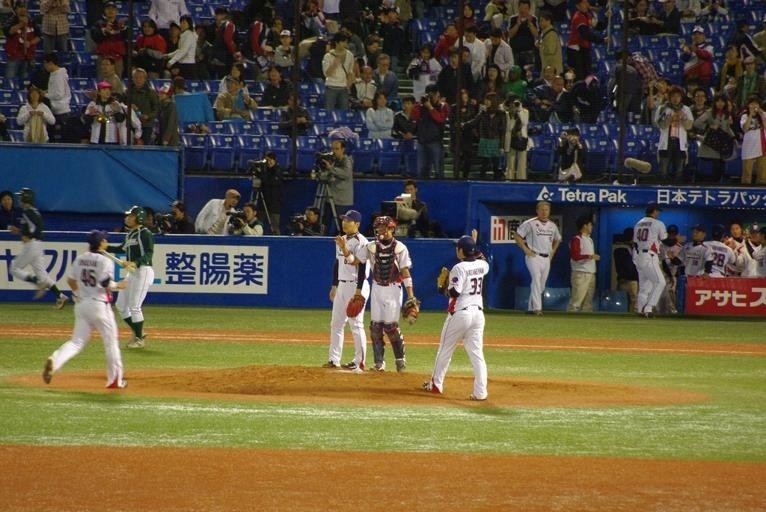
[{"x1": 421, "y1": 94, "x2": 433, "y2": 102}]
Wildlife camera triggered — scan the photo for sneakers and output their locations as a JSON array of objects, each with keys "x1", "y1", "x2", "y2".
[
  {"x1": 324, "y1": 361, "x2": 385, "y2": 370},
  {"x1": 127, "y1": 337, "x2": 144, "y2": 348},
  {"x1": 44, "y1": 359, "x2": 53, "y2": 384},
  {"x1": 55, "y1": 295, "x2": 68, "y2": 309}
]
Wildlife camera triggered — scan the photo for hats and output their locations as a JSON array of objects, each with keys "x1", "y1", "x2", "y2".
[
  {"x1": 280, "y1": 29, "x2": 291, "y2": 36},
  {"x1": 426, "y1": 85, "x2": 437, "y2": 92},
  {"x1": 511, "y1": 65, "x2": 521, "y2": 73},
  {"x1": 88, "y1": 230, "x2": 106, "y2": 246},
  {"x1": 459, "y1": 235, "x2": 476, "y2": 252},
  {"x1": 227, "y1": 76, "x2": 239, "y2": 84},
  {"x1": 693, "y1": 26, "x2": 704, "y2": 33},
  {"x1": 97, "y1": 82, "x2": 112, "y2": 87},
  {"x1": 647, "y1": 203, "x2": 661, "y2": 210},
  {"x1": 226, "y1": 190, "x2": 241, "y2": 198},
  {"x1": 170, "y1": 201, "x2": 184, "y2": 209},
  {"x1": 339, "y1": 210, "x2": 361, "y2": 221}
]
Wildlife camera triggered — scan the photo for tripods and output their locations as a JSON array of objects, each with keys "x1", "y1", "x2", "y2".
[
  {"x1": 314, "y1": 183, "x2": 342, "y2": 236},
  {"x1": 250, "y1": 191, "x2": 276, "y2": 235}
]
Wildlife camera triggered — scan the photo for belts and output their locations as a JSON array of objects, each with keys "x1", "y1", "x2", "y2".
[
  {"x1": 378, "y1": 283, "x2": 395, "y2": 286},
  {"x1": 535, "y1": 252, "x2": 548, "y2": 257}
]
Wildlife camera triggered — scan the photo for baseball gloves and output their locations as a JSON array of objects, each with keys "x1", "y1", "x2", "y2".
[
  {"x1": 403, "y1": 298, "x2": 421, "y2": 326},
  {"x1": 347, "y1": 295, "x2": 365, "y2": 317},
  {"x1": 437, "y1": 266, "x2": 449, "y2": 291}
]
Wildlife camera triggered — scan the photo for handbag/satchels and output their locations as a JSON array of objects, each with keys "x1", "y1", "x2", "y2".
[
  {"x1": 704, "y1": 129, "x2": 732, "y2": 155},
  {"x1": 202, "y1": 40, "x2": 223, "y2": 58},
  {"x1": 512, "y1": 137, "x2": 527, "y2": 151},
  {"x1": 559, "y1": 161, "x2": 582, "y2": 181},
  {"x1": 477, "y1": 138, "x2": 500, "y2": 157}
]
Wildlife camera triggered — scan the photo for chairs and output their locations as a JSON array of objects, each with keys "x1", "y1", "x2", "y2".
[{"x1": 0, "y1": 0, "x2": 765, "y2": 183}]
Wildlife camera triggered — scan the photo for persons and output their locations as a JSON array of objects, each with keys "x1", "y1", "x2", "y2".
[
  {"x1": 105, "y1": 205, "x2": 154, "y2": 349},
  {"x1": 42, "y1": 229, "x2": 128, "y2": 389},
  {"x1": 421, "y1": 228, "x2": 490, "y2": 400},
  {"x1": 322, "y1": 209, "x2": 371, "y2": 372},
  {"x1": 334, "y1": 214, "x2": 419, "y2": 373},
  {"x1": 0, "y1": 1, "x2": 766, "y2": 317}
]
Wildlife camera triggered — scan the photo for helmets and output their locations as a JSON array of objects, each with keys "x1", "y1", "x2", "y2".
[
  {"x1": 125, "y1": 206, "x2": 146, "y2": 224},
  {"x1": 373, "y1": 216, "x2": 396, "y2": 240},
  {"x1": 15, "y1": 188, "x2": 32, "y2": 201}
]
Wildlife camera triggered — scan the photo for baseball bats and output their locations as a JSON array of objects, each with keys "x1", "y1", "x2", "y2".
[{"x1": 103, "y1": 250, "x2": 136, "y2": 273}]
